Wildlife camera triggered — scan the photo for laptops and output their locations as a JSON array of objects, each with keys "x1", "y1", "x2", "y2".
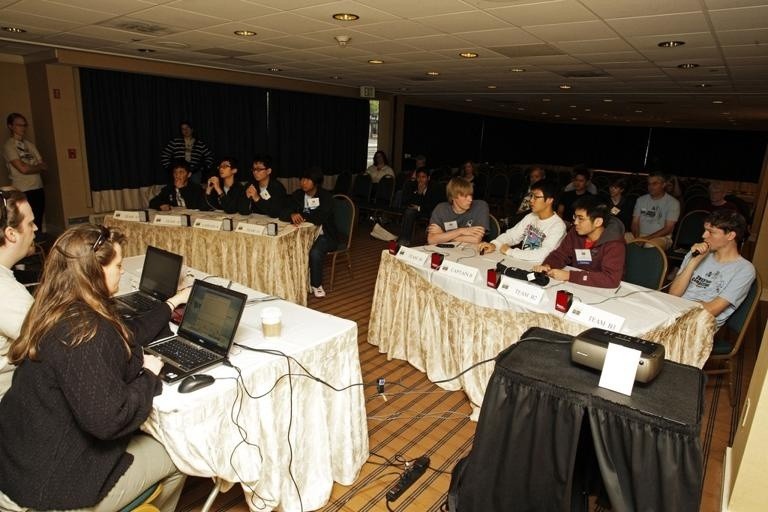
[
  {"x1": 110, "y1": 246, "x2": 183, "y2": 319},
  {"x1": 133, "y1": 279, "x2": 247, "y2": 383}
]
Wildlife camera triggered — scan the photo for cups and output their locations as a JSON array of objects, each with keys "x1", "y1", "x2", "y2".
[{"x1": 261, "y1": 314, "x2": 282, "y2": 338}]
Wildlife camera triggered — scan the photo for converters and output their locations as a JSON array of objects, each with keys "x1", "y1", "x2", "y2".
[{"x1": 414, "y1": 457, "x2": 429, "y2": 469}]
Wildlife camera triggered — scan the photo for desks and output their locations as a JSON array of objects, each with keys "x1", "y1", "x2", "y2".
[
  {"x1": 366, "y1": 242, "x2": 716, "y2": 423},
  {"x1": 103, "y1": 207, "x2": 315, "y2": 306},
  {"x1": 448, "y1": 327, "x2": 706, "y2": 511},
  {"x1": 111, "y1": 253, "x2": 371, "y2": 511}
]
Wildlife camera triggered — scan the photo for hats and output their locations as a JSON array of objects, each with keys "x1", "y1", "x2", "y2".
[{"x1": 530, "y1": 192, "x2": 553, "y2": 199}]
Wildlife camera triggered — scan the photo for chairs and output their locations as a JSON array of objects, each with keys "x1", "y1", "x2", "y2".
[
  {"x1": 483, "y1": 213, "x2": 501, "y2": 242},
  {"x1": 701, "y1": 270, "x2": 763, "y2": 408},
  {"x1": 624, "y1": 239, "x2": 668, "y2": 290},
  {"x1": 13, "y1": 238, "x2": 48, "y2": 289},
  {"x1": 674, "y1": 183, "x2": 713, "y2": 246},
  {"x1": 485, "y1": 166, "x2": 528, "y2": 218},
  {"x1": 624, "y1": 187, "x2": 649, "y2": 198},
  {"x1": 334, "y1": 170, "x2": 428, "y2": 241},
  {"x1": 437, "y1": 174, "x2": 452, "y2": 203},
  {"x1": 321, "y1": 195, "x2": 357, "y2": 290}
]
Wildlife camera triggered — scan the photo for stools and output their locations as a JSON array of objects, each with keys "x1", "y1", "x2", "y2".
[{"x1": 110, "y1": 484, "x2": 164, "y2": 511}]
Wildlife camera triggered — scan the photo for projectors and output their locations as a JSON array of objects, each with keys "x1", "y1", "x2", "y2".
[{"x1": 572, "y1": 327, "x2": 666, "y2": 384}]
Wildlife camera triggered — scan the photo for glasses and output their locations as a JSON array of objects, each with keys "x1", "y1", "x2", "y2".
[
  {"x1": 216, "y1": 165, "x2": 233, "y2": 170},
  {"x1": 572, "y1": 213, "x2": 594, "y2": 222}
]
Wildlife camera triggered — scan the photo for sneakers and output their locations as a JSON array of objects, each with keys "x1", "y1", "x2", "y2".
[{"x1": 312, "y1": 285, "x2": 326, "y2": 298}]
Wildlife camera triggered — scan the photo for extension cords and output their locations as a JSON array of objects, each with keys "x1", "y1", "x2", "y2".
[{"x1": 386, "y1": 455, "x2": 430, "y2": 501}]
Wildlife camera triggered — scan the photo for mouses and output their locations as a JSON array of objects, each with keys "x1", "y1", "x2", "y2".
[{"x1": 178, "y1": 372, "x2": 215, "y2": 392}]
